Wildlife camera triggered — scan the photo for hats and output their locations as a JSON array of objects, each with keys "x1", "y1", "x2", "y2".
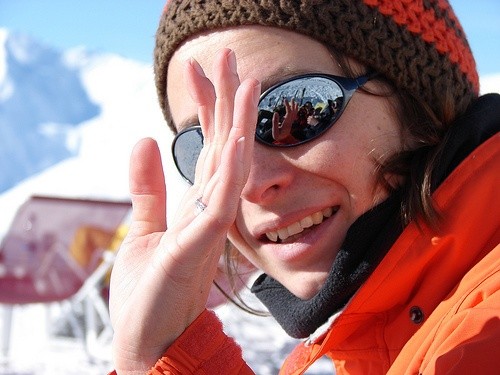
[{"x1": 153, "y1": 0, "x2": 480, "y2": 135}]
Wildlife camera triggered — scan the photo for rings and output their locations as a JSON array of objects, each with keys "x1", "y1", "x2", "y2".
[{"x1": 194, "y1": 199, "x2": 207, "y2": 211}]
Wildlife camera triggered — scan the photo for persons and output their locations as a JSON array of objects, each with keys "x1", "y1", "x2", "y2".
[{"x1": 108, "y1": 0, "x2": 500, "y2": 375}]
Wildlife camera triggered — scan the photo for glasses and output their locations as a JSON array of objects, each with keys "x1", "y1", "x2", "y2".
[{"x1": 171, "y1": 69, "x2": 379, "y2": 186}]
